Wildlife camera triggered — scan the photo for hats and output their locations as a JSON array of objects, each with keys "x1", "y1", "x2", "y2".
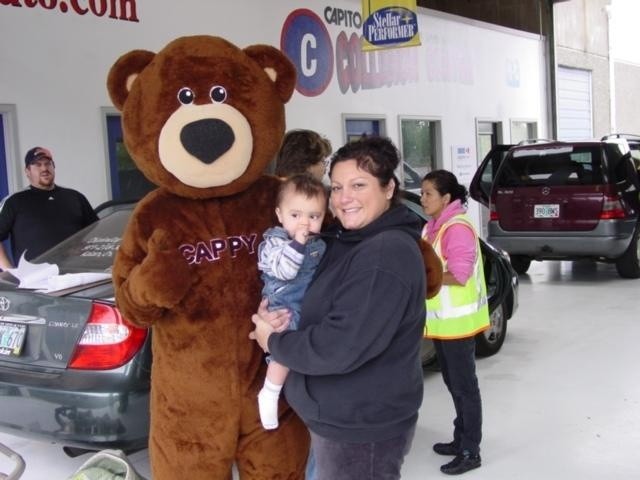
[{"x1": 25, "y1": 147, "x2": 52, "y2": 167}]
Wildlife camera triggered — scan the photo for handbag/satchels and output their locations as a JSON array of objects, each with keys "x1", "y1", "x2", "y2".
[{"x1": 479, "y1": 237, "x2": 513, "y2": 316}]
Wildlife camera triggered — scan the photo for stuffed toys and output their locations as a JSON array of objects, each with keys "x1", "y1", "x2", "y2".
[{"x1": 108, "y1": 36, "x2": 314, "y2": 480}]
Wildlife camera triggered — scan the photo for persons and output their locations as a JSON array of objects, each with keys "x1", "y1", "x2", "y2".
[
  {"x1": 254, "y1": 176, "x2": 329, "y2": 431},
  {"x1": 1, "y1": 147, "x2": 101, "y2": 272},
  {"x1": 273, "y1": 128, "x2": 332, "y2": 184},
  {"x1": 419, "y1": 170, "x2": 491, "y2": 477},
  {"x1": 248, "y1": 132, "x2": 428, "y2": 480}
]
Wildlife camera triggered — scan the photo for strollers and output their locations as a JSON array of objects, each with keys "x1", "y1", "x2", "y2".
[{"x1": 0, "y1": 444, "x2": 146, "y2": 480}]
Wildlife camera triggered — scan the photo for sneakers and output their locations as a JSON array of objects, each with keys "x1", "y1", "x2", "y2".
[
  {"x1": 440, "y1": 448, "x2": 481, "y2": 475},
  {"x1": 433, "y1": 440, "x2": 463, "y2": 455}
]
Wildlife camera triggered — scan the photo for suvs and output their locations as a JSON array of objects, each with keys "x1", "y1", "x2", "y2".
[{"x1": 471, "y1": 135, "x2": 640, "y2": 282}]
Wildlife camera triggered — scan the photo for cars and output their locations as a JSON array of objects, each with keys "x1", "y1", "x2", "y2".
[
  {"x1": 397, "y1": 165, "x2": 483, "y2": 235},
  {"x1": 0, "y1": 189, "x2": 518, "y2": 458}
]
